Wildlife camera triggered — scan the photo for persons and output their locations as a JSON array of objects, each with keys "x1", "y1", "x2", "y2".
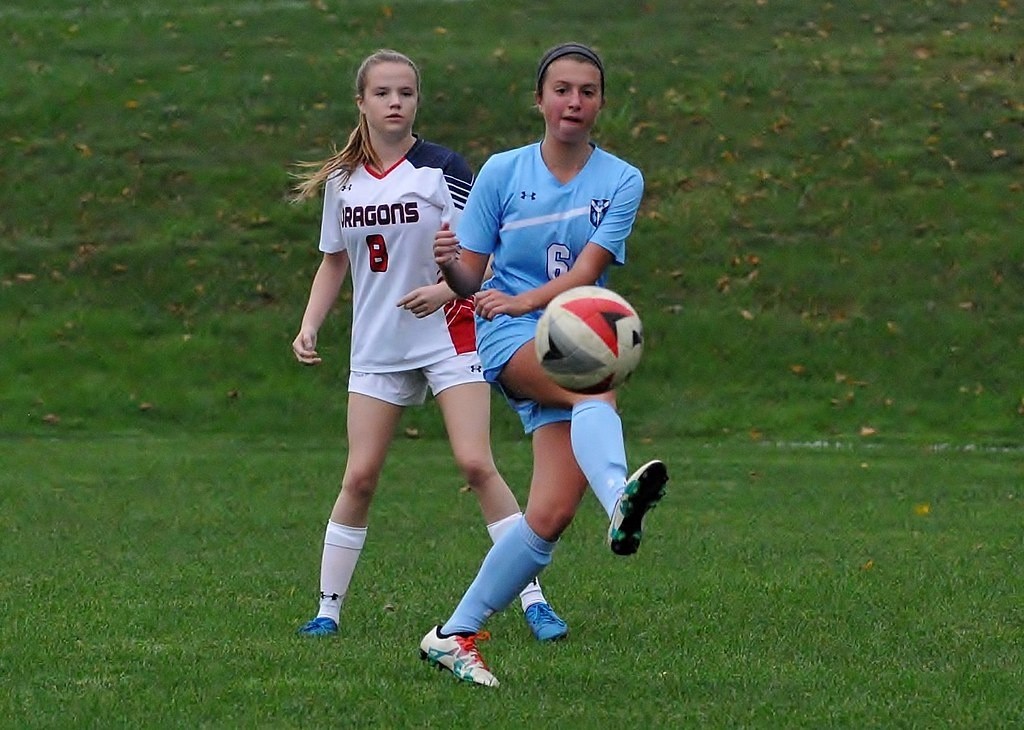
[
  {"x1": 419, "y1": 41, "x2": 669, "y2": 688},
  {"x1": 291, "y1": 48, "x2": 570, "y2": 640}
]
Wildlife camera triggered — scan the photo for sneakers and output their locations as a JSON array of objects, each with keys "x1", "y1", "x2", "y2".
[
  {"x1": 419, "y1": 624, "x2": 500, "y2": 690},
  {"x1": 524, "y1": 601, "x2": 569, "y2": 642},
  {"x1": 295, "y1": 617, "x2": 338, "y2": 636},
  {"x1": 606, "y1": 458, "x2": 668, "y2": 556}
]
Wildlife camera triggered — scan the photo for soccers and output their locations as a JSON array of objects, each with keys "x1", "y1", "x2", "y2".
[{"x1": 536, "y1": 284, "x2": 645, "y2": 394}]
